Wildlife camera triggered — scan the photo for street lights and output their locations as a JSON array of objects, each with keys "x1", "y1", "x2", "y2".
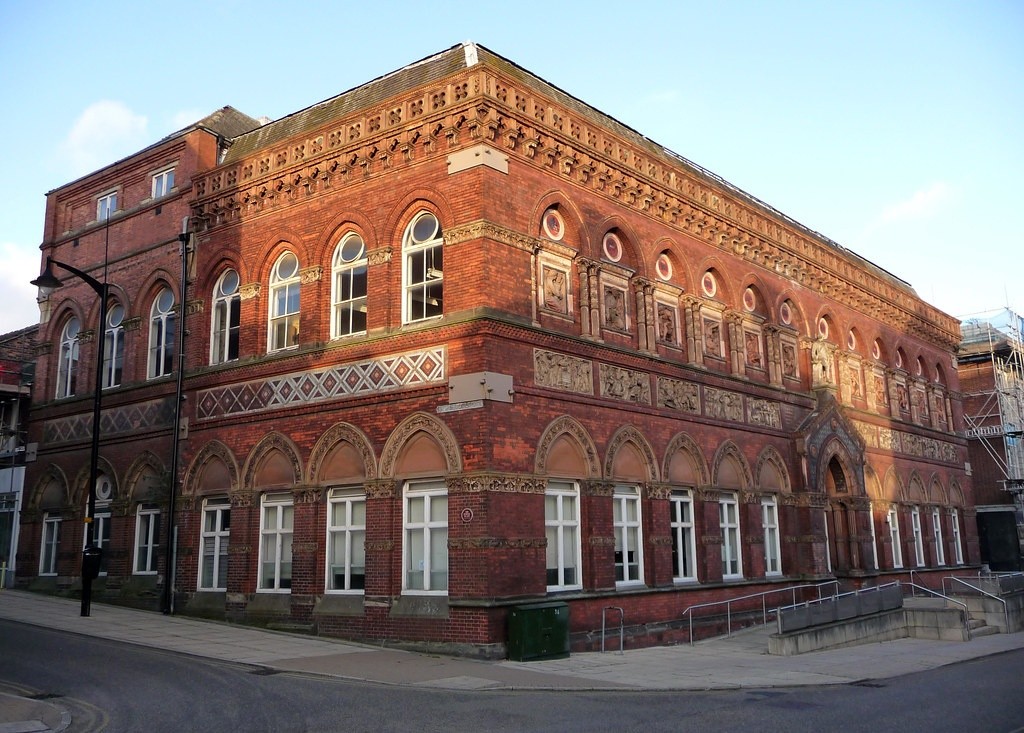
[{"x1": 30, "y1": 256, "x2": 108, "y2": 616}]
